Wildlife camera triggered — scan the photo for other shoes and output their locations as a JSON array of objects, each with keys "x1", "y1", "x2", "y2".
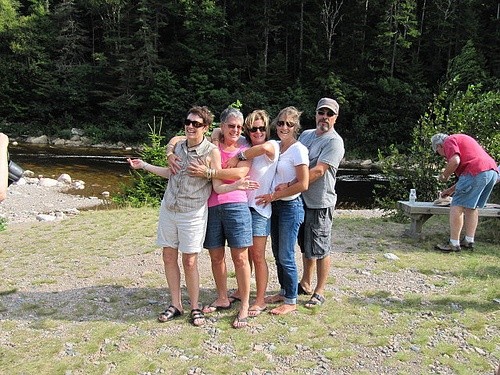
[
  {"x1": 435, "y1": 240, "x2": 462, "y2": 252},
  {"x1": 460, "y1": 237, "x2": 476, "y2": 249}
]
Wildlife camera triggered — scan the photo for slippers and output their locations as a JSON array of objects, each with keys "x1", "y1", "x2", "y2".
[
  {"x1": 202, "y1": 300, "x2": 232, "y2": 314},
  {"x1": 297, "y1": 283, "x2": 314, "y2": 296},
  {"x1": 232, "y1": 311, "x2": 250, "y2": 330},
  {"x1": 227, "y1": 292, "x2": 241, "y2": 303},
  {"x1": 304, "y1": 292, "x2": 327, "y2": 309},
  {"x1": 248, "y1": 305, "x2": 268, "y2": 318},
  {"x1": 158, "y1": 304, "x2": 184, "y2": 323},
  {"x1": 189, "y1": 308, "x2": 206, "y2": 327}
]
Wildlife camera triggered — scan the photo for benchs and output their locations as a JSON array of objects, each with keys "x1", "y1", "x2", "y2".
[{"x1": 396, "y1": 201, "x2": 500, "y2": 241}]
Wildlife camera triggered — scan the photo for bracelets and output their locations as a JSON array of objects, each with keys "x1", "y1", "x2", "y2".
[
  {"x1": 441, "y1": 175, "x2": 446, "y2": 179},
  {"x1": 166, "y1": 152, "x2": 173, "y2": 159},
  {"x1": 288, "y1": 181, "x2": 290, "y2": 187},
  {"x1": 238, "y1": 152, "x2": 248, "y2": 161},
  {"x1": 270, "y1": 192, "x2": 275, "y2": 201},
  {"x1": 213, "y1": 138, "x2": 220, "y2": 142},
  {"x1": 207, "y1": 169, "x2": 212, "y2": 181},
  {"x1": 142, "y1": 162, "x2": 149, "y2": 171}
]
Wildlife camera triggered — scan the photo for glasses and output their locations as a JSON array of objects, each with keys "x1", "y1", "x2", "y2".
[
  {"x1": 316, "y1": 108, "x2": 337, "y2": 118},
  {"x1": 276, "y1": 120, "x2": 296, "y2": 128},
  {"x1": 249, "y1": 125, "x2": 267, "y2": 134},
  {"x1": 227, "y1": 123, "x2": 243, "y2": 130},
  {"x1": 185, "y1": 118, "x2": 206, "y2": 129}
]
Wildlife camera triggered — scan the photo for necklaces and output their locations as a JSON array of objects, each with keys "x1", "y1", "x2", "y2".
[{"x1": 187, "y1": 141, "x2": 204, "y2": 148}]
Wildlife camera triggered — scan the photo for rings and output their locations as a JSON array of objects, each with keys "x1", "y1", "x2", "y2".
[{"x1": 263, "y1": 201, "x2": 265, "y2": 203}]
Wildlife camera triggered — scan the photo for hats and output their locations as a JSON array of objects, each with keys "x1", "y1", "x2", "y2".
[{"x1": 317, "y1": 97, "x2": 340, "y2": 115}]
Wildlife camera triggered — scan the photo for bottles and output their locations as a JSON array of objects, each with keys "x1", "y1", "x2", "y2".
[{"x1": 409, "y1": 189, "x2": 416, "y2": 203}]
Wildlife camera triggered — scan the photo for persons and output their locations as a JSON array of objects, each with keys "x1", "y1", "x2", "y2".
[
  {"x1": 431, "y1": 134, "x2": 500, "y2": 252},
  {"x1": 126, "y1": 98, "x2": 345, "y2": 328}
]
